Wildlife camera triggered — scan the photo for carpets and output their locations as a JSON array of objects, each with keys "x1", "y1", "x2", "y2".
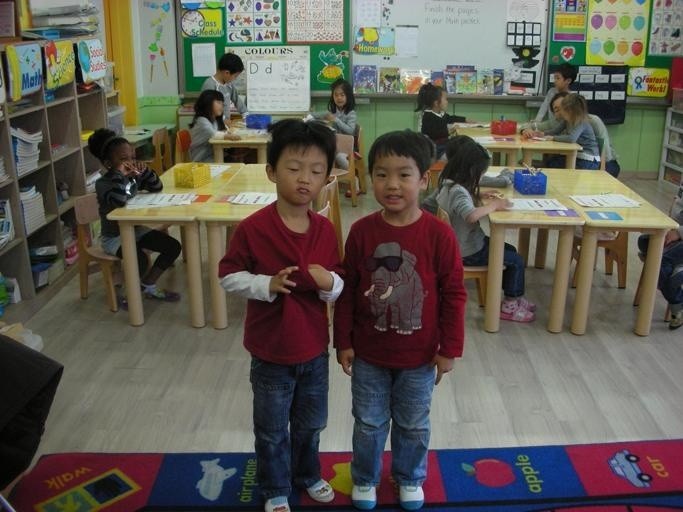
[{"x1": 3, "y1": 438, "x2": 683, "y2": 511}]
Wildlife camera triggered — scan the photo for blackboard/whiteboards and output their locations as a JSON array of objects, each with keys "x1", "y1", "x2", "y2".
[
  {"x1": 546, "y1": 1, "x2": 682, "y2": 101},
  {"x1": 351, "y1": 2, "x2": 552, "y2": 99},
  {"x1": 175, "y1": 2, "x2": 352, "y2": 98}
]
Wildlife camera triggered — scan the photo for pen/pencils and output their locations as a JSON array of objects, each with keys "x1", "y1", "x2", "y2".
[
  {"x1": 535, "y1": 122, "x2": 538, "y2": 131},
  {"x1": 522, "y1": 162, "x2": 541, "y2": 175}
]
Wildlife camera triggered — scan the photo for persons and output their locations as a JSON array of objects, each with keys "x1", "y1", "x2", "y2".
[
  {"x1": 436, "y1": 133, "x2": 538, "y2": 321},
  {"x1": 639, "y1": 182, "x2": 682, "y2": 331},
  {"x1": 520, "y1": 88, "x2": 620, "y2": 177},
  {"x1": 202, "y1": 53, "x2": 247, "y2": 128},
  {"x1": 218, "y1": 116, "x2": 345, "y2": 512},
  {"x1": 547, "y1": 93, "x2": 601, "y2": 169},
  {"x1": 414, "y1": 82, "x2": 479, "y2": 160},
  {"x1": 188, "y1": 90, "x2": 242, "y2": 163},
  {"x1": 422, "y1": 134, "x2": 515, "y2": 216},
  {"x1": 95, "y1": 135, "x2": 182, "y2": 311},
  {"x1": 334, "y1": 131, "x2": 468, "y2": 510},
  {"x1": 308, "y1": 78, "x2": 361, "y2": 198},
  {"x1": 532, "y1": 62, "x2": 579, "y2": 159},
  {"x1": 85, "y1": 126, "x2": 119, "y2": 192}
]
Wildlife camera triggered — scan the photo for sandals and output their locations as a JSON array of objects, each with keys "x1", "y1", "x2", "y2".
[{"x1": 116, "y1": 281, "x2": 182, "y2": 311}]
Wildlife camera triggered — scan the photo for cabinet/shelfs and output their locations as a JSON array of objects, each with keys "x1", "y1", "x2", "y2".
[
  {"x1": 0, "y1": 35, "x2": 108, "y2": 327},
  {"x1": 658, "y1": 106, "x2": 683, "y2": 188}
]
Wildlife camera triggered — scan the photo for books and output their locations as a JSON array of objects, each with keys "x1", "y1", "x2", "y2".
[
  {"x1": 353, "y1": 64, "x2": 510, "y2": 95},
  {"x1": 1, "y1": 124, "x2": 64, "y2": 294}
]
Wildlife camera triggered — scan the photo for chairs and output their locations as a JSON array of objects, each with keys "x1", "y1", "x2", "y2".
[
  {"x1": 74, "y1": 192, "x2": 122, "y2": 312},
  {"x1": 311, "y1": 121, "x2": 367, "y2": 262},
  {"x1": 143, "y1": 127, "x2": 172, "y2": 177},
  {"x1": 437, "y1": 206, "x2": 507, "y2": 308},
  {"x1": 429, "y1": 161, "x2": 448, "y2": 190}
]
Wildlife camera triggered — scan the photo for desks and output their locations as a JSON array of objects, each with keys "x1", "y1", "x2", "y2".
[
  {"x1": 106, "y1": 161, "x2": 279, "y2": 331},
  {"x1": 474, "y1": 168, "x2": 680, "y2": 337}
]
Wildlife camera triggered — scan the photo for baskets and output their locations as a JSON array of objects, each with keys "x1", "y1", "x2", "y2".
[
  {"x1": 174, "y1": 162, "x2": 211, "y2": 189},
  {"x1": 246, "y1": 114, "x2": 272, "y2": 129},
  {"x1": 514, "y1": 169, "x2": 547, "y2": 195},
  {"x1": 491, "y1": 119, "x2": 516, "y2": 135}
]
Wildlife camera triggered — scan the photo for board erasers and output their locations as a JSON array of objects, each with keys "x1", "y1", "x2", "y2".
[{"x1": 507, "y1": 90, "x2": 523, "y2": 95}]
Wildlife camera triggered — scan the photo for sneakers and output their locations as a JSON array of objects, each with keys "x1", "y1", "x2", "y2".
[
  {"x1": 501, "y1": 297, "x2": 537, "y2": 322},
  {"x1": 351, "y1": 484, "x2": 377, "y2": 510},
  {"x1": 307, "y1": 479, "x2": 335, "y2": 502},
  {"x1": 264, "y1": 496, "x2": 291, "y2": 511},
  {"x1": 398, "y1": 485, "x2": 425, "y2": 512},
  {"x1": 669, "y1": 309, "x2": 683, "y2": 329}
]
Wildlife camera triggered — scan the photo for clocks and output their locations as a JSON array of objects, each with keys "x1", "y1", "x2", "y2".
[{"x1": 181, "y1": 9, "x2": 205, "y2": 37}]
